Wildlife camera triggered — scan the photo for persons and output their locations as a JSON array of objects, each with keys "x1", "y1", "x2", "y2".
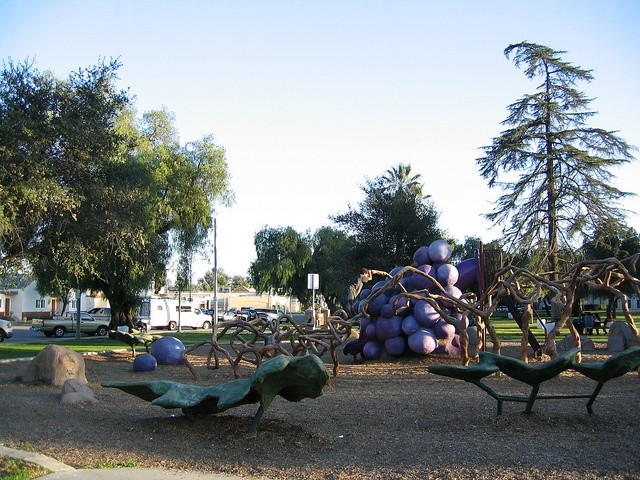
[
  {"x1": 248, "y1": 308, "x2": 256, "y2": 320},
  {"x1": 603, "y1": 313, "x2": 615, "y2": 335},
  {"x1": 584, "y1": 311, "x2": 594, "y2": 336},
  {"x1": 592, "y1": 313, "x2": 601, "y2": 336},
  {"x1": 264, "y1": 326, "x2": 290, "y2": 358},
  {"x1": 137, "y1": 319, "x2": 143, "y2": 332},
  {"x1": 343, "y1": 267, "x2": 372, "y2": 312}
]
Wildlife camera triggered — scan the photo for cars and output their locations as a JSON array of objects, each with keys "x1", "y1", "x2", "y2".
[
  {"x1": 87, "y1": 308, "x2": 112, "y2": 322},
  {"x1": 201, "y1": 307, "x2": 291, "y2": 325},
  {"x1": 0, "y1": 318, "x2": 13, "y2": 344}
]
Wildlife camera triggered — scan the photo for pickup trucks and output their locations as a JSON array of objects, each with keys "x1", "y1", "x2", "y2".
[{"x1": 31, "y1": 310, "x2": 111, "y2": 337}]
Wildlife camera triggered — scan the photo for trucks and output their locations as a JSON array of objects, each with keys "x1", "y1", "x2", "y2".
[{"x1": 137, "y1": 296, "x2": 213, "y2": 331}]
